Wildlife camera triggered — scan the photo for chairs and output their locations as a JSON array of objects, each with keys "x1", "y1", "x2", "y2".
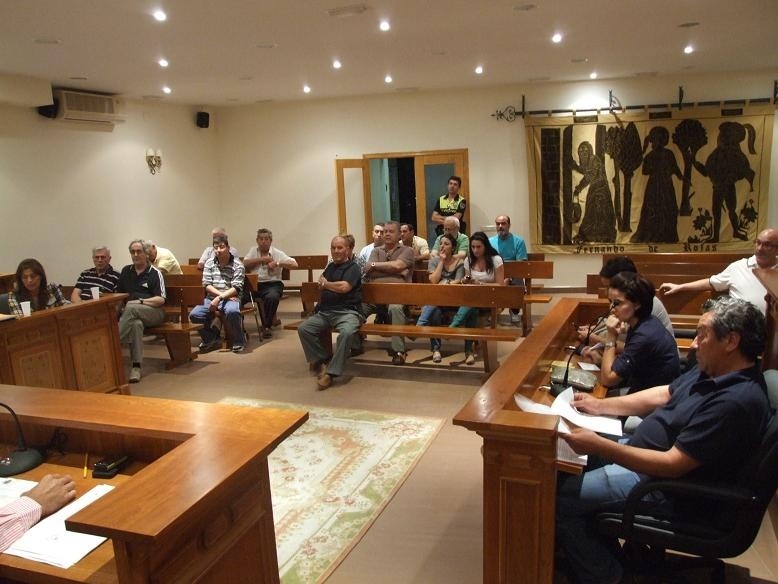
[{"x1": 595, "y1": 365, "x2": 778, "y2": 584}]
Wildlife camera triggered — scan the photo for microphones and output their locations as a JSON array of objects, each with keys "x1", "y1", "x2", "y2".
[
  {"x1": 0, "y1": 401, "x2": 44, "y2": 477},
  {"x1": 549, "y1": 302, "x2": 620, "y2": 394}
]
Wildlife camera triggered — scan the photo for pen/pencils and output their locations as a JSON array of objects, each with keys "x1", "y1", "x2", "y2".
[{"x1": 83, "y1": 450, "x2": 91, "y2": 479}]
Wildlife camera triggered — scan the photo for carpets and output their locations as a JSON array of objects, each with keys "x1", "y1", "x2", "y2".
[{"x1": 215, "y1": 397, "x2": 446, "y2": 584}]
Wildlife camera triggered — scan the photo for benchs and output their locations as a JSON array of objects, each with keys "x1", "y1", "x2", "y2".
[
  {"x1": 589, "y1": 250, "x2": 755, "y2": 315},
  {"x1": 140, "y1": 251, "x2": 551, "y2": 383}
]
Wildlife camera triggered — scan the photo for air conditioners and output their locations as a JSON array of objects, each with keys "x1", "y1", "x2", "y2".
[{"x1": 56, "y1": 91, "x2": 115, "y2": 121}]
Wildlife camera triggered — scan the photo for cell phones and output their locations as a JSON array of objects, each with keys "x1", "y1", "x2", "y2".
[{"x1": 92, "y1": 453, "x2": 128, "y2": 471}]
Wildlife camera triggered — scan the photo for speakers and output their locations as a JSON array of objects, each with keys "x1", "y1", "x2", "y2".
[{"x1": 196, "y1": 111, "x2": 211, "y2": 128}]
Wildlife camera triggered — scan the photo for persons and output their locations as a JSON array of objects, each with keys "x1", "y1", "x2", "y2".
[
  {"x1": 323, "y1": 234, "x2": 371, "y2": 355},
  {"x1": 658, "y1": 227, "x2": 778, "y2": 339},
  {"x1": 356, "y1": 220, "x2": 388, "y2": 323},
  {"x1": 143, "y1": 240, "x2": 185, "y2": 275},
  {"x1": 8, "y1": 256, "x2": 69, "y2": 322},
  {"x1": 116, "y1": 239, "x2": 168, "y2": 386},
  {"x1": 399, "y1": 223, "x2": 431, "y2": 264},
  {"x1": 0, "y1": 471, "x2": 80, "y2": 561},
  {"x1": 428, "y1": 215, "x2": 471, "y2": 261},
  {"x1": 241, "y1": 227, "x2": 300, "y2": 339},
  {"x1": 573, "y1": 257, "x2": 678, "y2": 364},
  {"x1": 361, "y1": 220, "x2": 415, "y2": 366},
  {"x1": 440, "y1": 231, "x2": 505, "y2": 366},
  {"x1": 550, "y1": 293, "x2": 772, "y2": 584},
  {"x1": 486, "y1": 213, "x2": 528, "y2": 325},
  {"x1": 404, "y1": 232, "x2": 466, "y2": 363},
  {"x1": 69, "y1": 244, "x2": 123, "y2": 320},
  {"x1": 583, "y1": 271, "x2": 682, "y2": 438},
  {"x1": 431, "y1": 175, "x2": 467, "y2": 232},
  {"x1": 296, "y1": 235, "x2": 365, "y2": 389},
  {"x1": 196, "y1": 226, "x2": 242, "y2": 274},
  {"x1": 188, "y1": 234, "x2": 247, "y2": 353}
]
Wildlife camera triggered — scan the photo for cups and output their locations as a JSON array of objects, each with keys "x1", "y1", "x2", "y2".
[
  {"x1": 20, "y1": 301, "x2": 31, "y2": 317},
  {"x1": 91, "y1": 287, "x2": 99, "y2": 300}
]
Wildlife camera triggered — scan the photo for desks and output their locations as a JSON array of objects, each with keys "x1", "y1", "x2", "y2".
[
  {"x1": 450, "y1": 296, "x2": 644, "y2": 584},
  {"x1": 0, "y1": 382, "x2": 311, "y2": 584},
  {"x1": 0, "y1": 290, "x2": 132, "y2": 396}
]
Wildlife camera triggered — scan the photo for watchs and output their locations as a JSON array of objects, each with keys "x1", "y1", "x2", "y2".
[{"x1": 138, "y1": 297, "x2": 144, "y2": 304}]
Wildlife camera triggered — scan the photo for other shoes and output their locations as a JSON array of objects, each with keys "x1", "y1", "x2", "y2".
[
  {"x1": 129, "y1": 367, "x2": 144, "y2": 384},
  {"x1": 198, "y1": 335, "x2": 217, "y2": 352},
  {"x1": 432, "y1": 349, "x2": 442, "y2": 362},
  {"x1": 232, "y1": 332, "x2": 247, "y2": 352},
  {"x1": 316, "y1": 361, "x2": 332, "y2": 391},
  {"x1": 404, "y1": 336, "x2": 415, "y2": 349},
  {"x1": 509, "y1": 308, "x2": 520, "y2": 323},
  {"x1": 350, "y1": 346, "x2": 364, "y2": 356},
  {"x1": 465, "y1": 353, "x2": 475, "y2": 365},
  {"x1": 263, "y1": 325, "x2": 273, "y2": 338},
  {"x1": 391, "y1": 351, "x2": 405, "y2": 365}
]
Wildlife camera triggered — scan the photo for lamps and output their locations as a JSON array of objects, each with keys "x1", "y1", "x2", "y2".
[{"x1": 146, "y1": 148, "x2": 163, "y2": 174}]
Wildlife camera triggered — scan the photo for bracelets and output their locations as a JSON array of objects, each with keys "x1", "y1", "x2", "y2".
[
  {"x1": 276, "y1": 261, "x2": 280, "y2": 268},
  {"x1": 218, "y1": 294, "x2": 224, "y2": 301},
  {"x1": 604, "y1": 340, "x2": 618, "y2": 348},
  {"x1": 371, "y1": 260, "x2": 376, "y2": 270},
  {"x1": 322, "y1": 279, "x2": 327, "y2": 288}
]
